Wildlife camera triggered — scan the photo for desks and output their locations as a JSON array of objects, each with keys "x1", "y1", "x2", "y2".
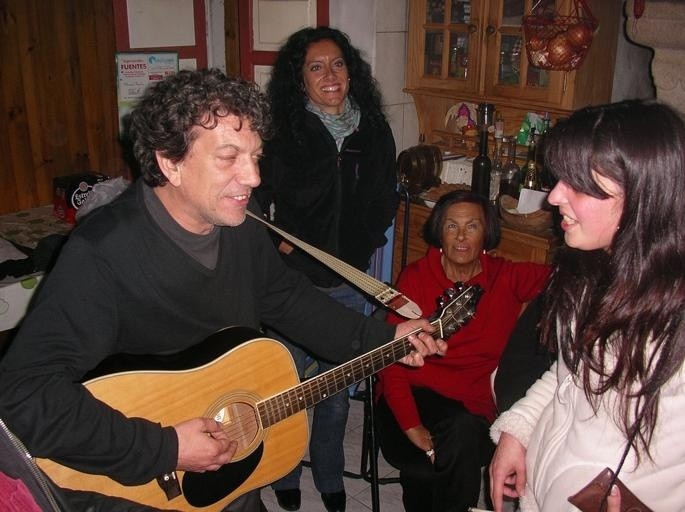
[
  {"x1": 0, "y1": 199, "x2": 78, "y2": 331},
  {"x1": 390, "y1": 181, "x2": 576, "y2": 311}
]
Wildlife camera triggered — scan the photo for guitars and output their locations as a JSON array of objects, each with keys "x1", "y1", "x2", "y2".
[{"x1": 33, "y1": 282, "x2": 484, "y2": 511}]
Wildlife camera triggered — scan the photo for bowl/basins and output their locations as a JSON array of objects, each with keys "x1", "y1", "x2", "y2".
[{"x1": 423, "y1": 200, "x2": 436, "y2": 208}]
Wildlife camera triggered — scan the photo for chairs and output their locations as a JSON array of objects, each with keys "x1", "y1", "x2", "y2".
[{"x1": 296, "y1": 295, "x2": 492, "y2": 512}]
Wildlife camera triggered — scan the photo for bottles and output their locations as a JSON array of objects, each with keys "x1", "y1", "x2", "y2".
[
  {"x1": 472, "y1": 119, "x2": 538, "y2": 206},
  {"x1": 450, "y1": 46, "x2": 466, "y2": 78},
  {"x1": 501, "y1": 51, "x2": 511, "y2": 81}
]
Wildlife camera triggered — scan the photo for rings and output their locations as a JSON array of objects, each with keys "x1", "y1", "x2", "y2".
[{"x1": 426, "y1": 448, "x2": 435, "y2": 456}]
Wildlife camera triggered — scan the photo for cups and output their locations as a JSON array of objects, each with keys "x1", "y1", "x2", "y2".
[{"x1": 477, "y1": 102, "x2": 494, "y2": 130}]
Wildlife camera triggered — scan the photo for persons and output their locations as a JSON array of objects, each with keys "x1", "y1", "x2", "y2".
[
  {"x1": 377, "y1": 190, "x2": 556, "y2": 512},
  {"x1": 251, "y1": 28, "x2": 398, "y2": 510},
  {"x1": 0, "y1": 73, "x2": 449, "y2": 511},
  {"x1": 486, "y1": 102, "x2": 685, "y2": 512}
]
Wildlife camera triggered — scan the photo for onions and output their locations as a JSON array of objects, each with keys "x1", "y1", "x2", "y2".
[{"x1": 529, "y1": 23, "x2": 592, "y2": 65}]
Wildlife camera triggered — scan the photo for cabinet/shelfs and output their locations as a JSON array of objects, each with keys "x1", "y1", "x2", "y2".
[{"x1": 404, "y1": 0, "x2": 614, "y2": 111}]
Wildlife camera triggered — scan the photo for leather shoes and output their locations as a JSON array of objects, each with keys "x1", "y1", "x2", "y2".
[
  {"x1": 321, "y1": 490, "x2": 346, "y2": 512},
  {"x1": 275, "y1": 489, "x2": 301, "y2": 511}
]
